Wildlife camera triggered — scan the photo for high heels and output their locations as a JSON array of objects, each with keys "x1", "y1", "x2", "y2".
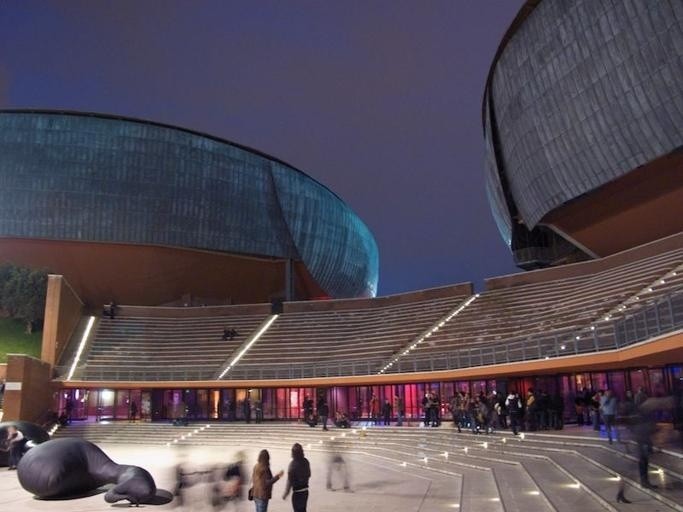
[{"x1": 616, "y1": 493, "x2": 632, "y2": 504}]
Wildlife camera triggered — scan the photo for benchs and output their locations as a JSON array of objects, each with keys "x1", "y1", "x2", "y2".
[{"x1": 81, "y1": 246, "x2": 683, "y2": 381}]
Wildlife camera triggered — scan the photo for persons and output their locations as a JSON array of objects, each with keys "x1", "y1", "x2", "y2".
[
  {"x1": 249, "y1": 449, "x2": 284, "y2": 511},
  {"x1": 58, "y1": 395, "x2": 73, "y2": 429},
  {"x1": 281, "y1": 443, "x2": 311, "y2": 512},
  {"x1": 108, "y1": 300, "x2": 116, "y2": 319},
  {"x1": 172, "y1": 408, "x2": 190, "y2": 426},
  {"x1": 219, "y1": 449, "x2": 246, "y2": 500},
  {"x1": 447, "y1": 387, "x2": 562, "y2": 436},
  {"x1": 615, "y1": 473, "x2": 631, "y2": 504},
  {"x1": 221, "y1": 326, "x2": 228, "y2": 342},
  {"x1": 324, "y1": 430, "x2": 355, "y2": 494},
  {"x1": 369, "y1": 392, "x2": 404, "y2": 426},
  {"x1": 228, "y1": 397, "x2": 262, "y2": 425},
  {"x1": 6, "y1": 423, "x2": 23, "y2": 469},
  {"x1": 621, "y1": 395, "x2": 659, "y2": 491},
  {"x1": 229, "y1": 328, "x2": 237, "y2": 338},
  {"x1": 0, "y1": 378, "x2": 4, "y2": 410},
  {"x1": 101, "y1": 308, "x2": 108, "y2": 316},
  {"x1": 95, "y1": 399, "x2": 104, "y2": 422},
  {"x1": 302, "y1": 389, "x2": 363, "y2": 430},
  {"x1": 574, "y1": 385, "x2": 681, "y2": 441},
  {"x1": 207, "y1": 464, "x2": 225, "y2": 509},
  {"x1": 130, "y1": 401, "x2": 139, "y2": 423},
  {"x1": 171, "y1": 452, "x2": 188, "y2": 506},
  {"x1": 422, "y1": 392, "x2": 441, "y2": 427}
]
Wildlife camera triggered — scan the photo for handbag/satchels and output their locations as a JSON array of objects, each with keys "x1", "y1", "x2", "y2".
[{"x1": 247, "y1": 488, "x2": 254, "y2": 501}]
